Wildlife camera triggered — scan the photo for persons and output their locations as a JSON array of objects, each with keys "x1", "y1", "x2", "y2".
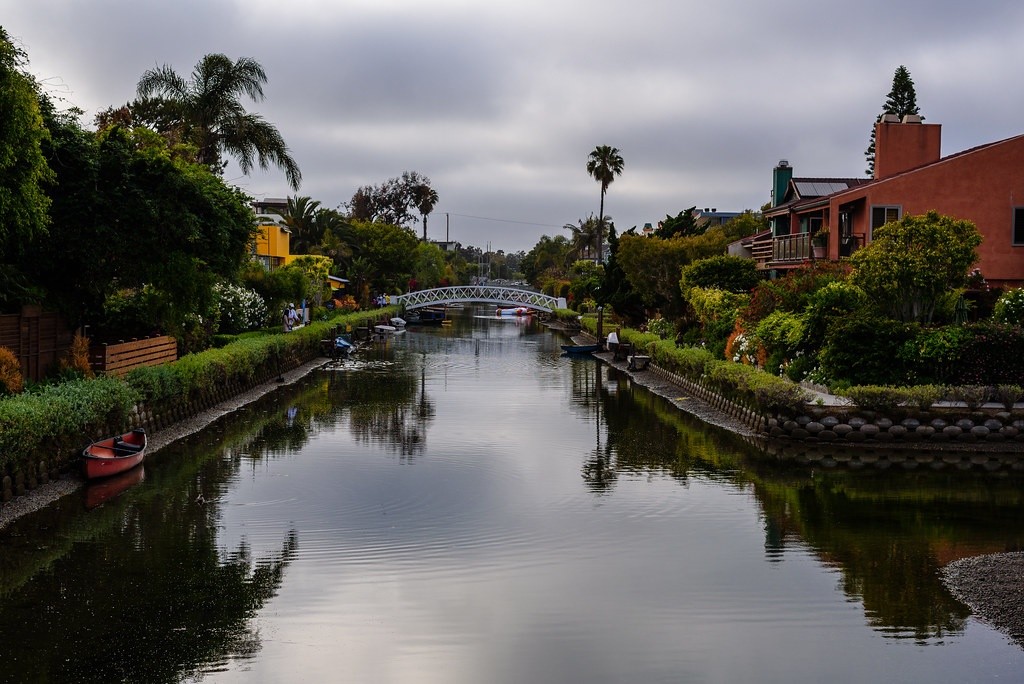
[
  {"x1": 287, "y1": 303, "x2": 300, "y2": 330},
  {"x1": 371, "y1": 292, "x2": 391, "y2": 307},
  {"x1": 282, "y1": 310, "x2": 291, "y2": 332}
]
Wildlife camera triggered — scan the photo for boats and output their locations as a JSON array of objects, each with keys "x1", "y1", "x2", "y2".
[
  {"x1": 560, "y1": 345, "x2": 601, "y2": 356},
  {"x1": 82, "y1": 428, "x2": 148, "y2": 479},
  {"x1": 404, "y1": 307, "x2": 448, "y2": 325},
  {"x1": 495, "y1": 308, "x2": 528, "y2": 314},
  {"x1": 497, "y1": 304, "x2": 526, "y2": 310}
]
